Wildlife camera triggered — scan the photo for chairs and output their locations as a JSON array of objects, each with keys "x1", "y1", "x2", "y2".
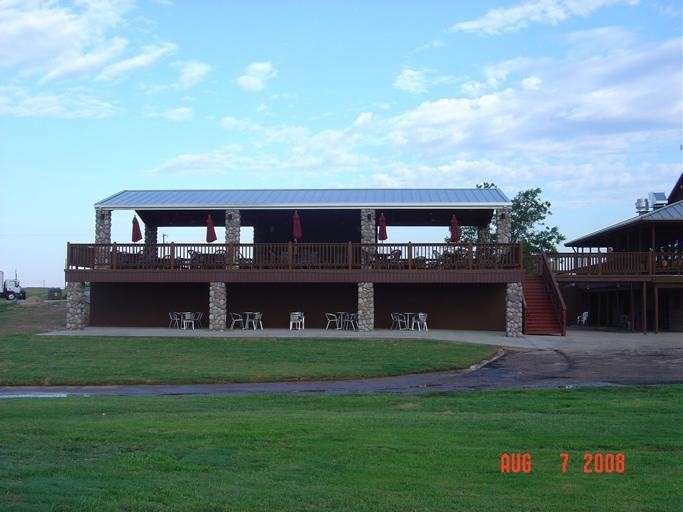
[
  {"x1": 168, "y1": 312, "x2": 428, "y2": 331},
  {"x1": 111, "y1": 251, "x2": 505, "y2": 270}
]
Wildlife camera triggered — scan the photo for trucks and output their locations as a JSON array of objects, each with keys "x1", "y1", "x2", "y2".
[{"x1": 0, "y1": 270, "x2": 21, "y2": 300}]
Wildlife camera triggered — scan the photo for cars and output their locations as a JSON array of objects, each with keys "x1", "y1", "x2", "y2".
[{"x1": 48, "y1": 288, "x2": 62, "y2": 299}]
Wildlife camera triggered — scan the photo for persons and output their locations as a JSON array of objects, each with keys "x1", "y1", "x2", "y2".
[{"x1": 18, "y1": 290, "x2": 25, "y2": 300}]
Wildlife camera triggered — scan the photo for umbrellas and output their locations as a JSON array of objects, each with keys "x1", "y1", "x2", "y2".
[
  {"x1": 292, "y1": 210, "x2": 302, "y2": 243},
  {"x1": 205, "y1": 214, "x2": 217, "y2": 243},
  {"x1": 131, "y1": 215, "x2": 143, "y2": 243},
  {"x1": 450, "y1": 213, "x2": 460, "y2": 243},
  {"x1": 377, "y1": 212, "x2": 387, "y2": 243}
]
